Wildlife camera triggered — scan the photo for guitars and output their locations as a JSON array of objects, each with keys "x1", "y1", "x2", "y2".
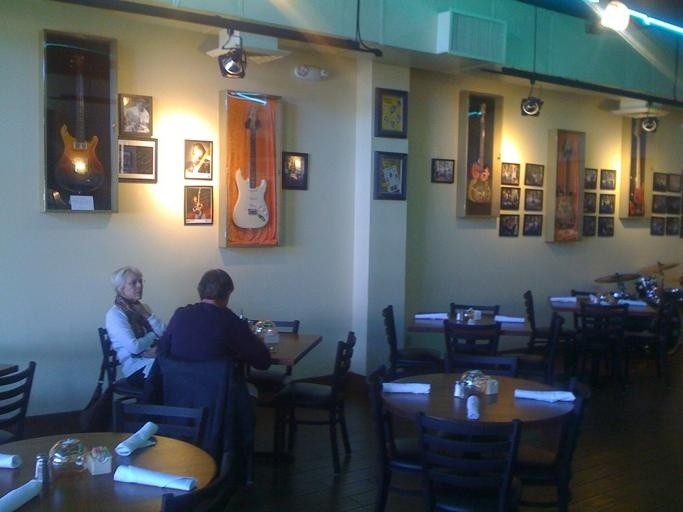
[
  {"x1": 232, "y1": 105, "x2": 270, "y2": 229},
  {"x1": 555, "y1": 138, "x2": 576, "y2": 229},
  {"x1": 467, "y1": 103, "x2": 492, "y2": 205},
  {"x1": 633, "y1": 119, "x2": 644, "y2": 204},
  {"x1": 54, "y1": 52, "x2": 106, "y2": 194}
]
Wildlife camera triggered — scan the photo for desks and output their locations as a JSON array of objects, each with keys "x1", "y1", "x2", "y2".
[
  {"x1": 0, "y1": 432, "x2": 218, "y2": 512},
  {"x1": 259, "y1": 334, "x2": 324, "y2": 435},
  {"x1": 1, "y1": 363, "x2": 19, "y2": 376}
]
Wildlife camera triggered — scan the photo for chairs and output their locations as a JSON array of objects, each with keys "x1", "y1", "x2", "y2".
[
  {"x1": 372, "y1": 290, "x2": 676, "y2": 512},
  {"x1": 159, "y1": 451, "x2": 239, "y2": 512},
  {"x1": 158, "y1": 354, "x2": 251, "y2": 490},
  {"x1": 109, "y1": 396, "x2": 209, "y2": 449},
  {"x1": 272, "y1": 329, "x2": 357, "y2": 474},
  {"x1": 247, "y1": 318, "x2": 302, "y2": 381},
  {"x1": 98, "y1": 326, "x2": 149, "y2": 401},
  {"x1": 1, "y1": 361, "x2": 37, "y2": 442}
]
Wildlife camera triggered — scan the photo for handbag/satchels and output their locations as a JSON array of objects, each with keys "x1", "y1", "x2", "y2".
[{"x1": 80, "y1": 384, "x2": 111, "y2": 432}]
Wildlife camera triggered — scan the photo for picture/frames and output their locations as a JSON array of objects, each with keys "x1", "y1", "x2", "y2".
[
  {"x1": 374, "y1": 87, "x2": 408, "y2": 139},
  {"x1": 184, "y1": 140, "x2": 213, "y2": 181},
  {"x1": 583, "y1": 167, "x2": 616, "y2": 237},
  {"x1": 118, "y1": 137, "x2": 158, "y2": 183},
  {"x1": 498, "y1": 162, "x2": 544, "y2": 237},
  {"x1": 184, "y1": 186, "x2": 213, "y2": 225},
  {"x1": 282, "y1": 151, "x2": 308, "y2": 190},
  {"x1": 373, "y1": 151, "x2": 408, "y2": 200},
  {"x1": 431, "y1": 158, "x2": 454, "y2": 183},
  {"x1": 118, "y1": 93, "x2": 153, "y2": 136},
  {"x1": 650, "y1": 172, "x2": 681, "y2": 236}
]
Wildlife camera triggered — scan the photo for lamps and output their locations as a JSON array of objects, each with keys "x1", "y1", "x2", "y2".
[
  {"x1": 640, "y1": 102, "x2": 658, "y2": 133},
  {"x1": 217, "y1": 28, "x2": 247, "y2": 79},
  {"x1": 520, "y1": 79, "x2": 544, "y2": 117}
]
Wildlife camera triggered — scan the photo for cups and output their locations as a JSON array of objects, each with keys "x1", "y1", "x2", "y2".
[{"x1": 473, "y1": 310, "x2": 481, "y2": 321}]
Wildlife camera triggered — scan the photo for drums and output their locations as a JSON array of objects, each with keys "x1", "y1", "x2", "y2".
[
  {"x1": 664, "y1": 288, "x2": 682, "y2": 303},
  {"x1": 635, "y1": 275, "x2": 664, "y2": 306},
  {"x1": 624, "y1": 299, "x2": 683, "y2": 356}
]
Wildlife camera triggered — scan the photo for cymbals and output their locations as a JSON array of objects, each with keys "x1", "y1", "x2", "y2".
[
  {"x1": 639, "y1": 262, "x2": 680, "y2": 273},
  {"x1": 595, "y1": 274, "x2": 639, "y2": 284}
]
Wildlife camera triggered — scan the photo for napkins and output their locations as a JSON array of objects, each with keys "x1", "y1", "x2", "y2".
[
  {"x1": 0, "y1": 451, "x2": 23, "y2": 471},
  {"x1": 114, "y1": 421, "x2": 159, "y2": 457},
  {"x1": 0, "y1": 479, "x2": 44, "y2": 512},
  {"x1": 113, "y1": 462, "x2": 198, "y2": 493}
]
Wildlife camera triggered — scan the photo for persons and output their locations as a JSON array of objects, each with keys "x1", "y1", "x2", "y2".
[
  {"x1": 192, "y1": 196, "x2": 206, "y2": 220},
  {"x1": 186, "y1": 145, "x2": 210, "y2": 174},
  {"x1": 584, "y1": 171, "x2": 614, "y2": 235},
  {"x1": 124, "y1": 99, "x2": 150, "y2": 133},
  {"x1": 388, "y1": 105, "x2": 398, "y2": 127},
  {"x1": 106, "y1": 266, "x2": 168, "y2": 386},
  {"x1": 290, "y1": 165, "x2": 299, "y2": 181},
  {"x1": 503, "y1": 164, "x2": 542, "y2": 235},
  {"x1": 141, "y1": 269, "x2": 270, "y2": 485}
]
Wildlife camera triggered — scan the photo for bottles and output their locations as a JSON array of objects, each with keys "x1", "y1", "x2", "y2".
[
  {"x1": 263, "y1": 324, "x2": 272, "y2": 336},
  {"x1": 453, "y1": 380, "x2": 461, "y2": 397},
  {"x1": 35, "y1": 455, "x2": 53, "y2": 490},
  {"x1": 456, "y1": 311, "x2": 471, "y2": 321}
]
